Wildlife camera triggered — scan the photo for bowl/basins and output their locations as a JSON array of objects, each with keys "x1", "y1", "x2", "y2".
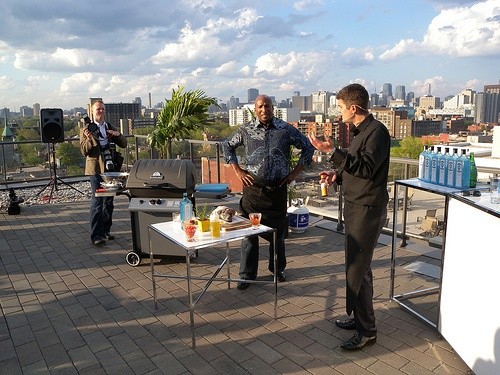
[{"x1": 100, "y1": 172, "x2": 129, "y2": 184}]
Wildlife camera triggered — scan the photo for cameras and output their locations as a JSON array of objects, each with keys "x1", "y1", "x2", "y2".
[{"x1": 83, "y1": 113, "x2": 105, "y2": 138}]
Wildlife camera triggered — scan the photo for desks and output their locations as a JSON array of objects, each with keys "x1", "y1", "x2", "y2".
[{"x1": 390, "y1": 177, "x2": 500, "y2": 375}]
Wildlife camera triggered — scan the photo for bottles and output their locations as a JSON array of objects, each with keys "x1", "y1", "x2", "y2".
[
  {"x1": 418, "y1": 145, "x2": 429, "y2": 181},
  {"x1": 424, "y1": 145, "x2": 436, "y2": 183},
  {"x1": 439, "y1": 148, "x2": 451, "y2": 186},
  {"x1": 494, "y1": 174, "x2": 500, "y2": 193},
  {"x1": 180, "y1": 193, "x2": 192, "y2": 221},
  {"x1": 469, "y1": 152, "x2": 477, "y2": 188},
  {"x1": 431, "y1": 147, "x2": 443, "y2": 184},
  {"x1": 446, "y1": 148, "x2": 459, "y2": 188},
  {"x1": 454, "y1": 149, "x2": 470, "y2": 190},
  {"x1": 321, "y1": 175, "x2": 328, "y2": 197}
]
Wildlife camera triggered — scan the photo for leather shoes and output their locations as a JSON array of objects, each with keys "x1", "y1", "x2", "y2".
[
  {"x1": 335, "y1": 319, "x2": 356, "y2": 329},
  {"x1": 339, "y1": 330, "x2": 376, "y2": 350},
  {"x1": 269, "y1": 269, "x2": 285, "y2": 282},
  {"x1": 238, "y1": 276, "x2": 250, "y2": 290}
]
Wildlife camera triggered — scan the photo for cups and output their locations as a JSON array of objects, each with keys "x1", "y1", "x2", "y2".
[
  {"x1": 249, "y1": 213, "x2": 261, "y2": 229},
  {"x1": 173, "y1": 213, "x2": 180, "y2": 222},
  {"x1": 186, "y1": 212, "x2": 194, "y2": 220},
  {"x1": 491, "y1": 178, "x2": 500, "y2": 204},
  {"x1": 181, "y1": 221, "x2": 198, "y2": 242},
  {"x1": 210, "y1": 221, "x2": 220, "y2": 238}
]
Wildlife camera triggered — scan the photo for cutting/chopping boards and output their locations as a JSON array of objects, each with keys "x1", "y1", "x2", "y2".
[{"x1": 221, "y1": 214, "x2": 252, "y2": 232}]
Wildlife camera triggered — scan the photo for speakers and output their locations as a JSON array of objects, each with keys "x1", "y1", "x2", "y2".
[
  {"x1": 39, "y1": 108, "x2": 65, "y2": 144},
  {"x1": 127, "y1": 157, "x2": 197, "y2": 198}
]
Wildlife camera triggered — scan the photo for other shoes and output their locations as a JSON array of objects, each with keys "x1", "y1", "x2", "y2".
[
  {"x1": 104, "y1": 232, "x2": 114, "y2": 240},
  {"x1": 94, "y1": 239, "x2": 106, "y2": 245}
]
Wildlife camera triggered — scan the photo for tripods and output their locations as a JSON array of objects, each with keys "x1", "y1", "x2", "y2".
[{"x1": 36, "y1": 143, "x2": 85, "y2": 201}]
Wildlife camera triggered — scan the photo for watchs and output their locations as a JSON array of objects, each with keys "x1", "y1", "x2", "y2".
[{"x1": 326, "y1": 148, "x2": 336, "y2": 157}]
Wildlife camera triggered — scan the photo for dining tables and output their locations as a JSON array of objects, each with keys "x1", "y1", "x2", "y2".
[{"x1": 147, "y1": 216, "x2": 277, "y2": 349}]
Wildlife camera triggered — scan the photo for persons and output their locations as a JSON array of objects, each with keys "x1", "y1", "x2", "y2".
[
  {"x1": 79, "y1": 99, "x2": 127, "y2": 246},
  {"x1": 307, "y1": 83, "x2": 392, "y2": 350},
  {"x1": 222, "y1": 97, "x2": 314, "y2": 289}
]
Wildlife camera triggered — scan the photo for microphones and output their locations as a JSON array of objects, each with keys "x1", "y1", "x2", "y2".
[{"x1": 341, "y1": 113, "x2": 354, "y2": 124}]
